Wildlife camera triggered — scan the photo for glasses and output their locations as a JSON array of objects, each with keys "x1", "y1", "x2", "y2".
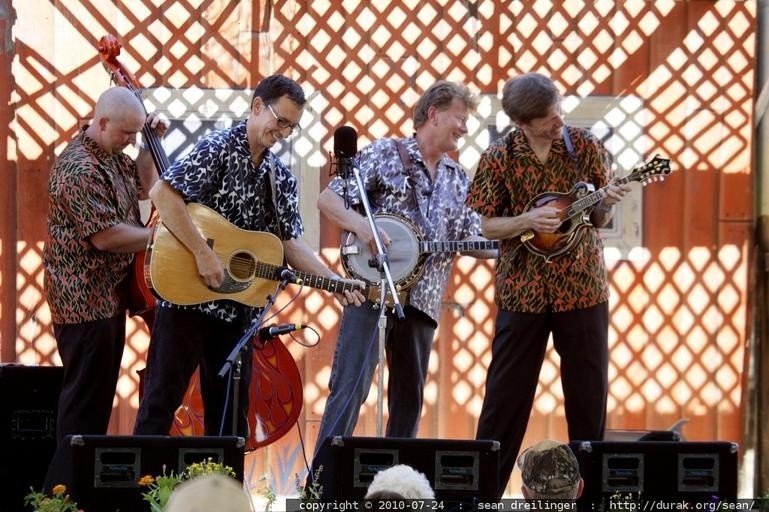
[{"x1": 266, "y1": 103, "x2": 303, "y2": 134}]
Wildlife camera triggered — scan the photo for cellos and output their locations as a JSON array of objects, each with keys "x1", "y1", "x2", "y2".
[{"x1": 97, "y1": 35, "x2": 303, "y2": 452}]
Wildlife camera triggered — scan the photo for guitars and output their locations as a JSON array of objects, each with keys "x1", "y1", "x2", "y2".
[{"x1": 144, "y1": 202, "x2": 407, "y2": 309}]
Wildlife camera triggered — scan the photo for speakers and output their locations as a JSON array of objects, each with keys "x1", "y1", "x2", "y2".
[
  {"x1": 570, "y1": 440, "x2": 738, "y2": 512},
  {"x1": 0, "y1": 368, "x2": 63, "y2": 512},
  {"x1": 323, "y1": 436, "x2": 500, "y2": 512},
  {"x1": 70, "y1": 433, "x2": 245, "y2": 511}
]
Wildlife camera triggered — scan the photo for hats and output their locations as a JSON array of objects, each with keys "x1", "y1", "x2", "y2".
[{"x1": 516, "y1": 440, "x2": 582, "y2": 499}]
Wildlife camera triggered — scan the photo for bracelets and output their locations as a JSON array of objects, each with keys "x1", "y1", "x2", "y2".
[{"x1": 597, "y1": 200, "x2": 613, "y2": 212}]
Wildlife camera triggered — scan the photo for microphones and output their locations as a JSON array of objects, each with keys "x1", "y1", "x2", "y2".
[
  {"x1": 260, "y1": 323, "x2": 307, "y2": 340},
  {"x1": 276, "y1": 266, "x2": 303, "y2": 284},
  {"x1": 329, "y1": 125, "x2": 362, "y2": 178}
]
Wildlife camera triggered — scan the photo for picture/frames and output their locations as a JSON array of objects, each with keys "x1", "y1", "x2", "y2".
[
  {"x1": 132, "y1": 88, "x2": 322, "y2": 269},
  {"x1": 458, "y1": 94, "x2": 643, "y2": 261}
]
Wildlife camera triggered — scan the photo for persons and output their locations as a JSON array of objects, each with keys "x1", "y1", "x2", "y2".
[
  {"x1": 159, "y1": 474, "x2": 253, "y2": 512},
  {"x1": 360, "y1": 461, "x2": 436, "y2": 512},
  {"x1": 45, "y1": 87, "x2": 166, "y2": 500},
  {"x1": 132, "y1": 74, "x2": 365, "y2": 439},
  {"x1": 463, "y1": 73, "x2": 631, "y2": 507},
  {"x1": 515, "y1": 439, "x2": 584, "y2": 512},
  {"x1": 303, "y1": 78, "x2": 501, "y2": 501}
]
